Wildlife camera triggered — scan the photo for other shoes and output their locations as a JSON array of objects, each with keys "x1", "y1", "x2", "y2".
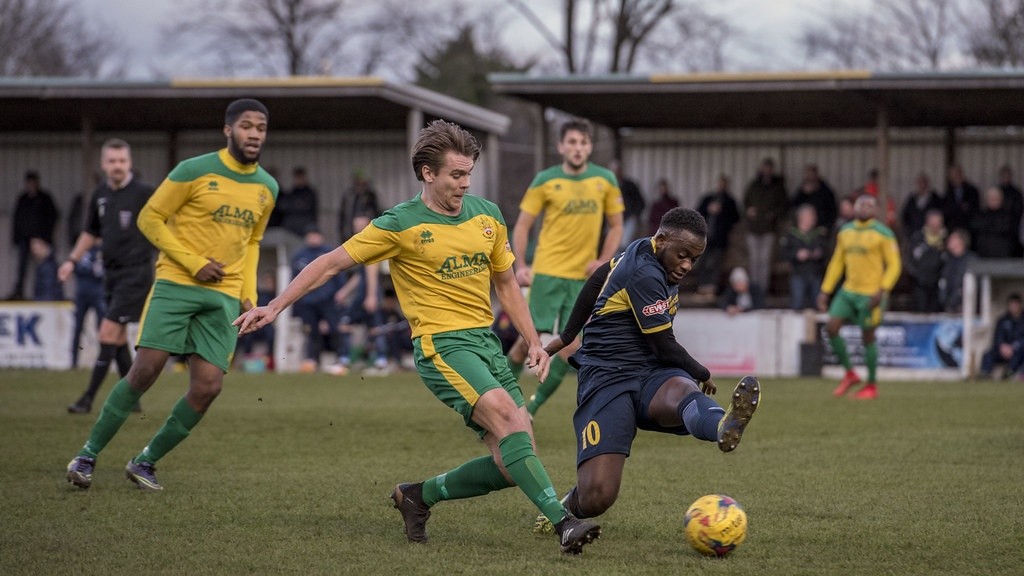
[
  {"x1": 855, "y1": 384, "x2": 877, "y2": 400},
  {"x1": 68, "y1": 399, "x2": 91, "y2": 413},
  {"x1": 834, "y1": 373, "x2": 860, "y2": 396}
]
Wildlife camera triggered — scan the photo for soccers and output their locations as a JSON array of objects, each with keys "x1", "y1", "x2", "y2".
[{"x1": 683, "y1": 494, "x2": 749, "y2": 557}]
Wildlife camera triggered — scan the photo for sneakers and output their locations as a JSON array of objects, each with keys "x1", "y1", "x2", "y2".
[
  {"x1": 717, "y1": 376, "x2": 762, "y2": 452},
  {"x1": 67, "y1": 456, "x2": 96, "y2": 488},
  {"x1": 390, "y1": 481, "x2": 431, "y2": 544},
  {"x1": 125, "y1": 457, "x2": 163, "y2": 489},
  {"x1": 559, "y1": 513, "x2": 602, "y2": 554},
  {"x1": 534, "y1": 512, "x2": 555, "y2": 537}
]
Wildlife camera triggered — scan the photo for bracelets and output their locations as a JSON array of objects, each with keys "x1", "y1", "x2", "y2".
[{"x1": 64, "y1": 257, "x2": 77, "y2": 265}]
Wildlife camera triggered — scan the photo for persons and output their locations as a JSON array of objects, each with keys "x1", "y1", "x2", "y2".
[
  {"x1": 242, "y1": 162, "x2": 413, "y2": 374},
  {"x1": 232, "y1": 118, "x2": 601, "y2": 555},
  {"x1": 504, "y1": 119, "x2": 625, "y2": 424},
  {"x1": 6, "y1": 170, "x2": 106, "y2": 369},
  {"x1": 601, "y1": 154, "x2": 1024, "y2": 381},
  {"x1": 532, "y1": 205, "x2": 762, "y2": 536},
  {"x1": 56, "y1": 137, "x2": 167, "y2": 412},
  {"x1": 815, "y1": 194, "x2": 902, "y2": 399},
  {"x1": 65, "y1": 98, "x2": 279, "y2": 492}
]
